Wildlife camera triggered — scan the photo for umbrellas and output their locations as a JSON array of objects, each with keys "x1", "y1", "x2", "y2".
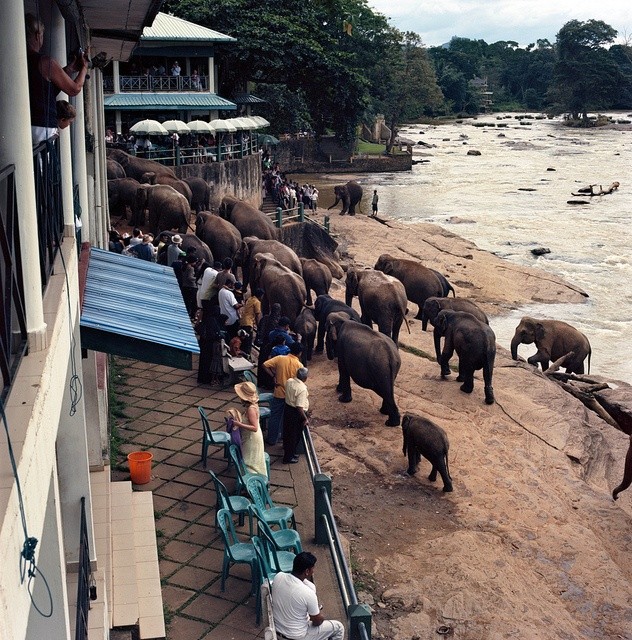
[
  {"x1": 226, "y1": 117, "x2": 251, "y2": 132},
  {"x1": 238, "y1": 114, "x2": 260, "y2": 130},
  {"x1": 162, "y1": 118, "x2": 190, "y2": 136},
  {"x1": 246, "y1": 112, "x2": 266, "y2": 128},
  {"x1": 128, "y1": 118, "x2": 168, "y2": 159},
  {"x1": 255, "y1": 113, "x2": 270, "y2": 127},
  {"x1": 257, "y1": 133, "x2": 281, "y2": 149},
  {"x1": 208, "y1": 118, "x2": 238, "y2": 142},
  {"x1": 186, "y1": 118, "x2": 215, "y2": 139}
]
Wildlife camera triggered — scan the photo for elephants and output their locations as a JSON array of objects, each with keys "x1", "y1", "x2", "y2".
[
  {"x1": 217, "y1": 193, "x2": 278, "y2": 241},
  {"x1": 138, "y1": 170, "x2": 194, "y2": 209},
  {"x1": 237, "y1": 234, "x2": 304, "y2": 295},
  {"x1": 431, "y1": 308, "x2": 498, "y2": 406},
  {"x1": 103, "y1": 178, "x2": 147, "y2": 228},
  {"x1": 364, "y1": 253, "x2": 457, "y2": 322},
  {"x1": 322, "y1": 311, "x2": 404, "y2": 429},
  {"x1": 150, "y1": 230, "x2": 215, "y2": 270},
  {"x1": 193, "y1": 210, "x2": 242, "y2": 282},
  {"x1": 344, "y1": 266, "x2": 412, "y2": 349},
  {"x1": 510, "y1": 316, "x2": 592, "y2": 376},
  {"x1": 295, "y1": 306, "x2": 318, "y2": 363},
  {"x1": 181, "y1": 176, "x2": 211, "y2": 214},
  {"x1": 398, "y1": 412, "x2": 453, "y2": 492},
  {"x1": 106, "y1": 158, "x2": 126, "y2": 180},
  {"x1": 312, "y1": 293, "x2": 362, "y2": 353},
  {"x1": 106, "y1": 148, "x2": 177, "y2": 184},
  {"x1": 130, "y1": 182, "x2": 195, "y2": 234},
  {"x1": 328, "y1": 179, "x2": 364, "y2": 216},
  {"x1": 296, "y1": 254, "x2": 334, "y2": 307},
  {"x1": 418, "y1": 296, "x2": 489, "y2": 332},
  {"x1": 248, "y1": 251, "x2": 315, "y2": 331}
]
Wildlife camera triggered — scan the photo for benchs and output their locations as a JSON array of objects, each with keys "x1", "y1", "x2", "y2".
[
  {"x1": 220, "y1": 338, "x2": 254, "y2": 382},
  {"x1": 261, "y1": 577, "x2": 277, "y2": 640}
]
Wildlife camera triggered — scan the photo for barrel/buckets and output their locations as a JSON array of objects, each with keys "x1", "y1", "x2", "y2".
[{"x1": 127, "y1": 451, "x2": 153, "y2": 485}]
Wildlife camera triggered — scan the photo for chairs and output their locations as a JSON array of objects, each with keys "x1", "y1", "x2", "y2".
[
  {"x1": 244, "y1": 371, "x2": 273, "y2": 407},
  {"x1": 198, "y1": 407, "x2": 231, "y2": 471},
  {"x1": 246, "y1": 475, "x2": 297, "y2": 552},
  {"x1": 260, "y1": 407, "x2": 270, "y2": 431},
  {"x1": 229, "y1": 444, "x2": 266, "y2": 508},
  {"x1": 265, "y1": 451, "x2": 270, "y2": 480},
  {"x1": 258, "y1": 521, "x2": 297, "y2": 573},
  {"x1": 249, "y1": 503, "x2": 303, "y2": 554},
  {"x1": 217, "y1": 509, "x2": 260, "y2": 597},
  {"x1": 208, "y1": 469, "x2": 253, "y2": 543},
  {"x1": 252, "y1": 536, "x2": 277, "y2": 625}
]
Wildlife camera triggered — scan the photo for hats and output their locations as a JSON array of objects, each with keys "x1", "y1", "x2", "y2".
[
  {"x1": 188, "y1": 256, "x2": 197, "y2": 264},
  {"x1": 234, "y1": 382, "x2": 259, "y2": 402},
  {"x1": 142, "y1": 234, "x2": 153, "y2": 245},
  {"x1": 171, "y1": 234, "x2": 183, "y2": 244}
]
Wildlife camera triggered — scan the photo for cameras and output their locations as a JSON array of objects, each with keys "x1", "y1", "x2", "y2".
[{"x1": 75, "y1": 45, "x2": 90, "y2": 79}]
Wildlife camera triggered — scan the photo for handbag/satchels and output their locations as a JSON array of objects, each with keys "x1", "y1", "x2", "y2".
[{"x1": 254, "y1": 331, "x2": 265, "y2": 347}]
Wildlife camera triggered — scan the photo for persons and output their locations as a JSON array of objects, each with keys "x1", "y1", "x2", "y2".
[
  {"x1": 229, "y1": 329, "x2": 253, "y2": 363},
  {"x1": 262, "y1": 341, "x2": 304, "y2": 445},
  {"x1": 282, "y1": 366, "x2": 311, "y2": 463},
  {"x1": 260, "y1": 315, "x2": 302, "y2": 391},
  {"x1": 90, "y1": 52, "x2": 111, "y2": 71},
  {"x1": 169, "y1": 133, "x2": 251, "y2": 168},
  {"x1": 132, "y1": 133, "x2": 154, "y2": 153},
  {"x1": 143, "y1": 63, "x2": 168, "y2": 88},
  {"x1": 171, "y1": 59, "x2": 182, "y2": 80},
  {"x1": 109, "y1": 227, "x2": 220, "y2": 312},
  {"x1": 269, "y1": 551, "x2": 344, "y2": 640},
  {"x1": 223, "y1": 281, "x2": 243, "y2": 303},
  {"x1": 49, "y1": 93, "x2": 80, "y2": 133},
  {"x1": 24, "y1": 10, "x2": 92, "y2": 116},
  {"x1": 104, "y1": 127, "x2": 113, "y2": 144},
  {"x1": 240, "y1": 284, "x2": 263, "y2": 355},
  {"x1": 257, "y1": 145, "x2": 322, "y2": 215},
  {"x1": 268, "y1": 335, "x2": 290, "y2": 358},
  {"x1": 222, "y1": 258, "x2": 235, "y2": 282},
  {"x1": 218, "y1": 273, "x2": 242, "y2": 344},
  {"x1": 74, "y1": 205, "x2": 84, "y2": 231},
  {"x1": 209, "y1": 311, "x2": 229, "y2": 387},
  {"x1": 255, "y1": 302, "x2": 282, "y2": 388},
  {"x1": 295, "y1": 129, "x2": 311, "y2": 140},
  {"x1": 370, "y1": 189, "x2": 379, "y2": 216},
  {"x1": 230, "y1": 380, "x2": 267, "y2": 477},
  {"x1": 191, "y1": 68, "x2": 204, "y2": 92}
]
